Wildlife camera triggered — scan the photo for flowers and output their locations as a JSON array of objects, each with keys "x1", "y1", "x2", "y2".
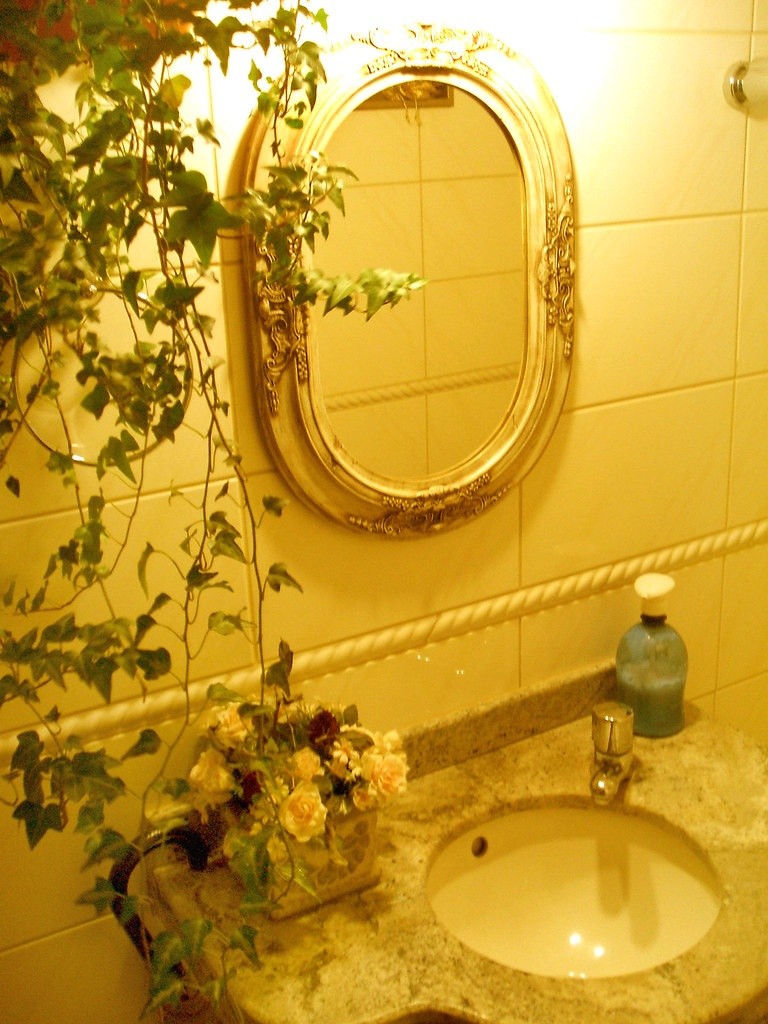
[{"x1": 188, "y1": 694, "x2": 411, "y2": 860}]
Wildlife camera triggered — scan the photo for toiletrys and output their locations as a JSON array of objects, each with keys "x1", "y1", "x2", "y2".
[{"x1": 615, "y1": 572, "x2": 689, "y2": 739}]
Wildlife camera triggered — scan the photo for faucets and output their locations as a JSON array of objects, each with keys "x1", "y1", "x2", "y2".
[{"x1": 588, "y1": 701, "x2": 635, "y2": 807}]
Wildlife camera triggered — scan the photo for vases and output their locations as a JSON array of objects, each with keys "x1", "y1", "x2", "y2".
[{"x1": 222, "y1": 808, "x2": 377, "y2": 921}]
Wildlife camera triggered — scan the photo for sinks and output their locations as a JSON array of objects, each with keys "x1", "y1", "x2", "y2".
[{"x1": 422, "y1": 794, "x2": 721, "y2": 979}]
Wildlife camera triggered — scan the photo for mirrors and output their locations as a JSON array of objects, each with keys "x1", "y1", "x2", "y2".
[{"x1": 242, "y1": 16, "x2": 575, "y2": 540}]
